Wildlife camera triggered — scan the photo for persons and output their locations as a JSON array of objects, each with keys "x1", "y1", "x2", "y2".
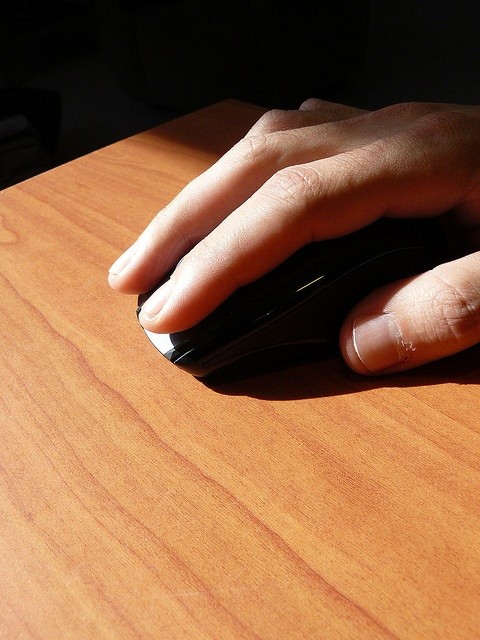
[{"x1": 107, "y1": 98, "x2": 480, "y2": 381}]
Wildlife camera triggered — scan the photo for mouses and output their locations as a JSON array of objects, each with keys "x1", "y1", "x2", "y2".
[{"x1": 135, "y1": 207, "x2": 480, "y2": 380}]
